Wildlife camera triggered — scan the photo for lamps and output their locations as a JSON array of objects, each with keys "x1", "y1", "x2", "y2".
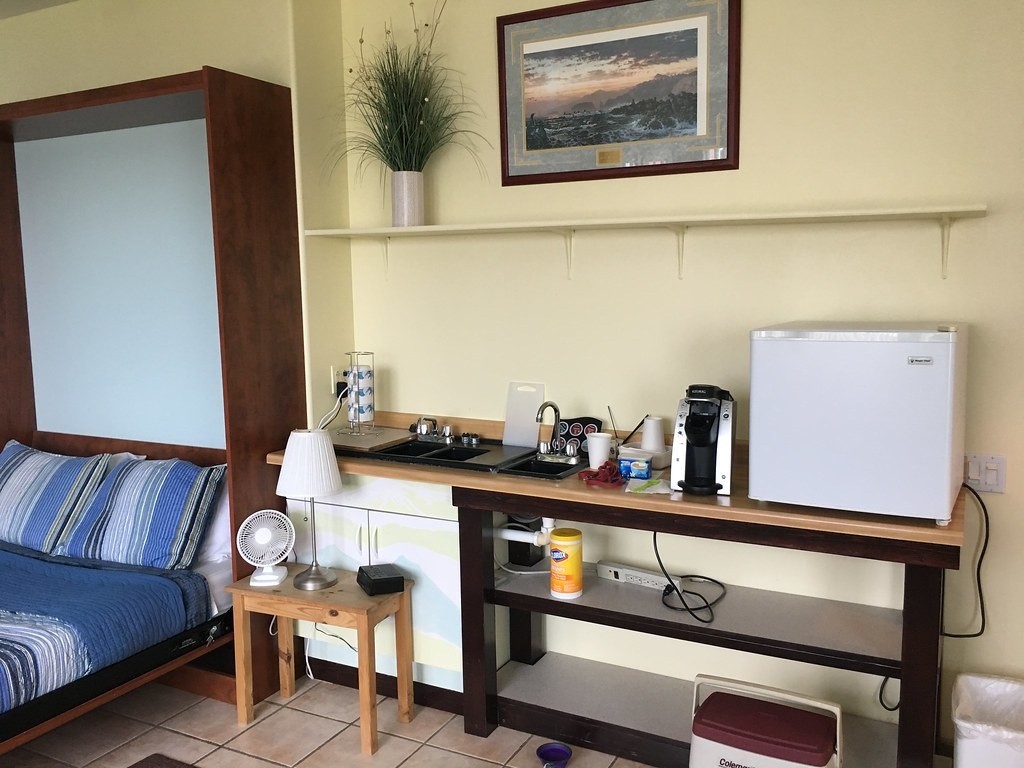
[{"x1": 275, "y1": 430, "x2": 343, "y2": 591}]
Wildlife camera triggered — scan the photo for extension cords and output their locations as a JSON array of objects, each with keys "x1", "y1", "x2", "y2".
[{"x1": 596, "y1": 560, "x2": 684, "y2": 595}]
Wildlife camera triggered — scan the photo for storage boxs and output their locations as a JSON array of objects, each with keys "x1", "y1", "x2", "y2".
[{"x1": 689, "y1": 674, "x2": 845, "y2": 768}]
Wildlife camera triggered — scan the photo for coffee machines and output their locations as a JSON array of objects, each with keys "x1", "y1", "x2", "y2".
[{"x1": 670, "y1": 384, "x2": 737, "y2": 496}]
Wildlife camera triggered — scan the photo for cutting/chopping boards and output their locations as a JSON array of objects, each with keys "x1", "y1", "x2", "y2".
[{"x1": 502, "y1": 382, "x2": 544, "y2": 448}]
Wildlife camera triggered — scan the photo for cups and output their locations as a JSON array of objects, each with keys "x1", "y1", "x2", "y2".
[
  {"x1": 587, "y1": 433, "x2": 612, "y2": 469},
  {"x1": 347, "y1": 365, "x2": 373, "y2": 424},
  {"x1": 535, "y1": 742, "x2": 572, "y2": 768},
  {"x1": 641, "y1": 417, "x2": 666, "y2": 452}
]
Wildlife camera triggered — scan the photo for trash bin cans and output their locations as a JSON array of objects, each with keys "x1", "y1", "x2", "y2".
[{"x1": 951, "y1": 673, "x2": 1024, "y2": 768}]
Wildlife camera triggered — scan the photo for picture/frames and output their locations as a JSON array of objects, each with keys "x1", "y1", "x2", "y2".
[{"x1": 495, "y1": 0, "x2": 742, "y2": 187}]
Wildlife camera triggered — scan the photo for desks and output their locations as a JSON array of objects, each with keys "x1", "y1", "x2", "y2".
[{"x1": 224, "y1": 561, "x2": 416, "y2": 756}]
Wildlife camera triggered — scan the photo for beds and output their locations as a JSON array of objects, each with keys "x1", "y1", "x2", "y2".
[{"x1": 0, "y1": 428, "x2": 309, "y2": 755}]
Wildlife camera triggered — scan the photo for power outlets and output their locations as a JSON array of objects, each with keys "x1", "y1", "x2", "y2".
[{"x1": 330, "y1": 365, "x2": 349, "y2": 394}]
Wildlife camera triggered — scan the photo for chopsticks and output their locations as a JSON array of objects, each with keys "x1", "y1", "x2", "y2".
[{"x1": 607, "y1": 406, "x2": 649, "y2": 444}]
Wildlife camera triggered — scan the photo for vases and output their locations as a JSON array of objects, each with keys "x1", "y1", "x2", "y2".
[{"x1": 392, "y1": 171, "x2": 425, "y2": 228}]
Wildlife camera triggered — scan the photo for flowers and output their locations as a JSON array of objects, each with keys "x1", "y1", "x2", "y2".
[{"x1": 317, "y1": 0, "x2": 495, "y2": 211}]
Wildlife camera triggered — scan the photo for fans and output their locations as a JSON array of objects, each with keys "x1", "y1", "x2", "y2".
[{"x1": 236, "y1": 509, "x2": 296, "y2": 587}]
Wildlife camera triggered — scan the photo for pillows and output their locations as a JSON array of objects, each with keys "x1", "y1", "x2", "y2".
[
  {"x1": 50, "y1": 457, "x2": 230, "y2": 571},
  {"x1": 105, "y1": 452, "x2": 147, "y2": 477},
  {"x1": 196, "y1": 463, "x2": 231, "y2": 565},
  {"x1": 0, "y1": 439, "x2": 111, "y2": 557}
]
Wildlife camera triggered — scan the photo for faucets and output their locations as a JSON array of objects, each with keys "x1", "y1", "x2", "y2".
[
  {"x1": 536, "y1": 400, "x2": 580, "y2": 464},
  {"x1": 417, "y1": 416, "x2": 454, "y2": 444}
]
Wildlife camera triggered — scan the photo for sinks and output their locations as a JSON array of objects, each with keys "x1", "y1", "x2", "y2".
[
  {"x1": 498, "y1": 451, "x2": 590, "y2": 480},
  {"x1": 375, "y1": 440, "x2": 449, "y2": 463},
  {"x1": 418, "y1": 444, "x2": 490, "y2": 463}
]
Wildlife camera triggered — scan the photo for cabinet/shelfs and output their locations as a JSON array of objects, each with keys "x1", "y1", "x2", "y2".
[{"x1": 264, "y1": 204, "x2": 989, "y2": 768}]
